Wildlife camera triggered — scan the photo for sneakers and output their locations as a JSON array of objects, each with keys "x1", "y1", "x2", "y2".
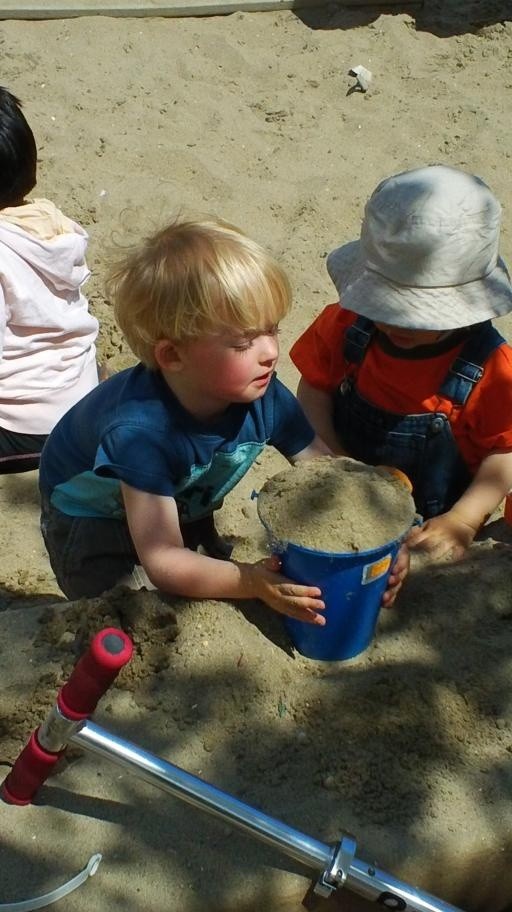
[{"x1": 114, "y1": 586, "x2": 179, "y2": 642}]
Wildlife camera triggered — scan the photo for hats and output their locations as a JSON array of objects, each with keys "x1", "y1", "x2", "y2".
[{"x1": 326, "y1": 165, "x2": 511, "y2": 330}]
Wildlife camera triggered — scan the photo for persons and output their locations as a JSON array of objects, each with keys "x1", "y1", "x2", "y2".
[
  {"x1": 33, "y1": 218, "x2": 410, "y2": 630},
  {"x1": 283, "y1": 165, "x2": 510, "y2": 562},
  {"x1": 0, "y1": 83, "x2": 102, "y2": 479}
]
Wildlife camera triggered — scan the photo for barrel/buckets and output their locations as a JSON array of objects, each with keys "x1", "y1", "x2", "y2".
[{"x1": 255, "y1": 460, "x2": 424, "y2": 661}]
